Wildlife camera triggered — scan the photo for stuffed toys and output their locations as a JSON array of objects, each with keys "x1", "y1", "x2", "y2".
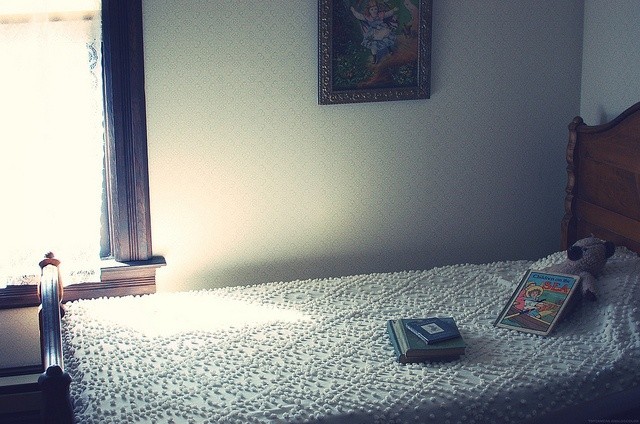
[{"x1": 542, "y1": 235, "x2": 615, "y2": 299}]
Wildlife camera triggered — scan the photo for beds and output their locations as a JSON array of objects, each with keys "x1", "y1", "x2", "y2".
[{"x1": 39, "y1": 102, "x2": 640, "y2": 424}]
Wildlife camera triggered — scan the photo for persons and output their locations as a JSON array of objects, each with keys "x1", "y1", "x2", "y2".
[{"x1": 348, "y1": 2, "x2": 397, "y2": 63}]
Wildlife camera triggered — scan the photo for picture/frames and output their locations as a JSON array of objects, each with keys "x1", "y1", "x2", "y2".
[{"x1": 318, "y1": 1, "x2": 432, "y2": 105}]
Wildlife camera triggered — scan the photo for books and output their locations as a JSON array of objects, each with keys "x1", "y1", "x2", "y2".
[
  {"x1": 387, "y1": 317, "x2": 466, "y2": 363},
  {"x1": 495, "y1": 270, "x2": 581, "y2": 338}
]
